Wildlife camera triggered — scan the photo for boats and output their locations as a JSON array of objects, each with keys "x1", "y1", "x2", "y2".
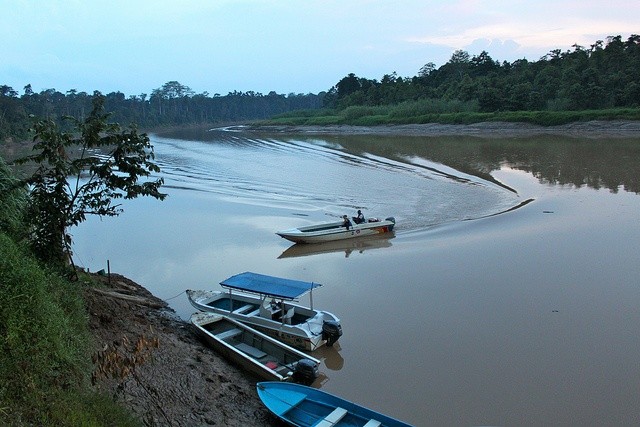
[
  {"x1": 191, "y1": 312, "x2": 321, "y2": 386},
  {"x1": 186, "y1": 272, "x2": 343, "y2": 351},
  {"x1": 256, "y1": 381, "x2": 417, "y2": 427},
  {"x1": 275, "y1": 217, "x2": 395, "y2": 243}
]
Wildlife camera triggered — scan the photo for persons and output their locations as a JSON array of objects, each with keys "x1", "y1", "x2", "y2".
[
  {"x1": 353, "y1": 210, "x2": 365, "y2": 224},
  {"x1": 341, "y1": 215, "x2": 352, "y2": 229}
]
